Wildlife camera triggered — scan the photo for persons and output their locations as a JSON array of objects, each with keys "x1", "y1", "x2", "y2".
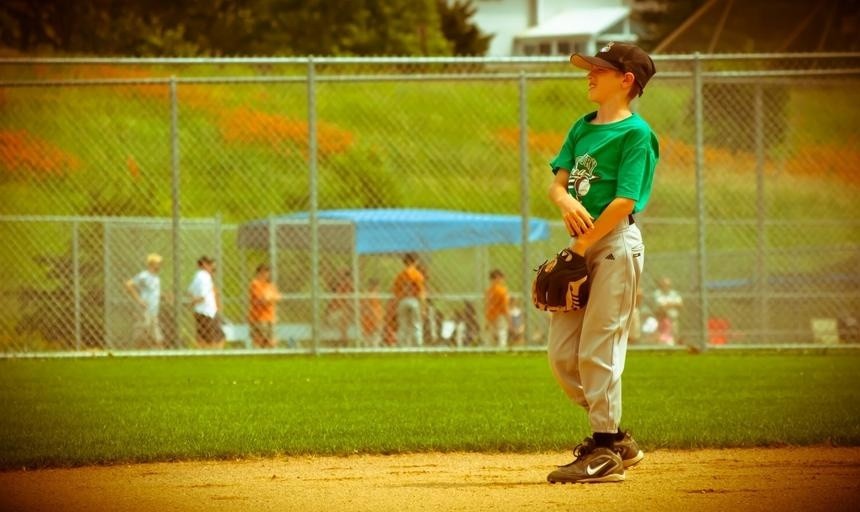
[
  {"x1": 161, "y1": 284, "x2": 181, "y2": 355},
  {"x1": 124, "y1": 252, "x2": 165, "y2": 349},
  {"x1": 246, "y1": 263, "x2": 279, "y2": 349},
  {"x1": 188, "y1": 252, "x2": 226, "y2": 350},
  {"x1": 531, "y1": 40, "x2": 664, "y2": 486}
]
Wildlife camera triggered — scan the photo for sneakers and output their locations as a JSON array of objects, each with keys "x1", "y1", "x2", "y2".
[
  {"x1": 580, "y1": 429, "x2": 643, "y2": 469},
  {"x1": 545, "y1": 447, "x2": 627, "y2": 482}
]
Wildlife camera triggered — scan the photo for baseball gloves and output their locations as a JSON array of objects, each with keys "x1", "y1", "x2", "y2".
[{"x1": 530, "y1": 248, "x2": 588, "y2": 315}]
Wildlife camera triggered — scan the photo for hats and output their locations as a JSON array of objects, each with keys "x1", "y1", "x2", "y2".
[
  {"x1": 570, "y1": 41, "x2": 656, "y2": 98},
  {"x1": 197, "y1": 256, "x2": 216, "y2": 267},
  {"x1": 144, "y1": 252, "x2": 162, "y2": 266},
  {"x1": 258, "y1": 262, "x2": 271, "y2": 273}
]
